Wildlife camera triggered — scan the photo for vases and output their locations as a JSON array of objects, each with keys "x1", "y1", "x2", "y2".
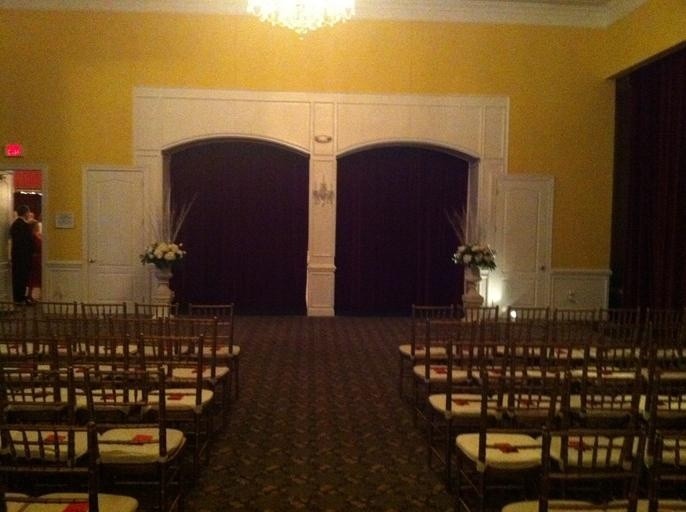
[
  {"x1": 461, "y1": 269, "x2": 485, "y2": 322},
  {"x1": 152, "y1": 265, "x2": 174, "y2": 321}
]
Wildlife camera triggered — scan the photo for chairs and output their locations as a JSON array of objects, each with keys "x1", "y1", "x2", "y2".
[
  {"x1": 578, "y1": 321, "x2": 647, "y2": 393},
  {"x1": 77, "y1": 328, "x2": 143, "y2": 425},
  {"x1": 493, "y1": 339, "x2": 563, "y2": 428},
  {"x1": 5, "y1": 327, "x2": 86, "y2": 427},
  {"x1": 537, "y1": 370, "x2": 644, "y2": 502},
  {"x1": 34, "y1": 301, "x2": 87, "y2": 359},
  {"x1": 188, "y1": 303, "x2": 241, "y2": 403},
  {"x1": 84, "y1": 366, "x2": 186, "y2": 512},
  {"x1": 486, "y1": 305, "x2": 552, "y2": 365},
  {"x1": 534, "y1": 324, "x2": 591, "y2": 392},
  {"x1": 34, "y1": 317, "x2": 74, "y2": 386},
  {"x1": 171, "y1": 315, "x2": 230, "y2": 435},
  {"x1": 411, "y1": 319, "x2": 479, "y2": 428},
  {"x1": 0, "y1": 299, "x2": 34, "y2": 359},
  {"x1": 134, "y1": 301, "x2": 193, "y2": 361},
  {"x1": 446, "y1": 303, "x2": 499, "y2": 366},
  {"x1": 114, "y1": 318, "x2": 169, "y2": 389},
  {"x1": 500, "y1": 426, "x2": 646, "y2": 512},
  {"x1": 0, "y1": 327, "x2": 16, "y2": 426},
  {"x1": 634, "y1": 307, "x2": 686, "y2": 366},
  {"x1": 641, "y1": 321, "x2": 686, "y2": 395},
  {"x1": 643, "y1": 375, "x2": 686, "y2": 498},
  {"x1": 0, "y1": 492, "x2": 32, "y2": 512},
  {"x1": 397, "y1": 303, "x2": 455, "y2": 401},
  {"x1": 638, "y1": 343, "x2": 686, "y2": 432},
  {"x1": 557, "y1": 343, "x2": 641, "y2": 428},
  {"x1": 74, "y1": 317, "x2": 114, "y2": 389},
  {"x1": 635, "y1": 439, "x2": 686, "y2": 512},
  {"x1": 0, "y1": 317, "x2": 35, "y2": 387},
  {"x1": 580, "y1": 307, "x2": 642, "y2": 364},
  {"x1": 139, "y1": 332, "x2": 214, "y2": 479},
  {"x1": 422, "y1": 338, "x2": 505, "y2": 492},
  {"x1": 454, "y1": 371, "x2": 542, "y2": 512},
  {"x1": 0, "y1": 422, "x2": 140, "y2": 512},
  {"x1": 0, "y1": 366, "x2": 101, "y2": 495},
  {"x1": 479, "y1": 320, "x2": 541, "y2": 392},
  {"x1": 81, "y1": 302, "x2": 137, "y2": 361},
  {"x1": 645, "y1": 426, "x2": 686, "y2": 498},
  {"x1": 537, "y1": 307, "x2": 596, "y2": 366}
]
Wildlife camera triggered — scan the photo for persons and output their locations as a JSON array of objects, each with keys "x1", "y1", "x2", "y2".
[
  {"x1": 25, "y1": 207, "x2": 42, "y2": 303},
  {"x1": 10, "y1": 204, "x2": 35, "y2": 307}
]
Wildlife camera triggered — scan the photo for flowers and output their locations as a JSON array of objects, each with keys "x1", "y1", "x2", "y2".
[
  {"x1": 444, "y1": 205, "x2": 498, "y2": 276},
  {"x1": 140, "y1": 200, "x2": 192, "y2": 266}
]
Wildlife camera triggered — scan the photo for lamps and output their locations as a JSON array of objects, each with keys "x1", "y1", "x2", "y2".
[{"x1": 246, "y1": 0, "x2": 357, "y2": 34}]
